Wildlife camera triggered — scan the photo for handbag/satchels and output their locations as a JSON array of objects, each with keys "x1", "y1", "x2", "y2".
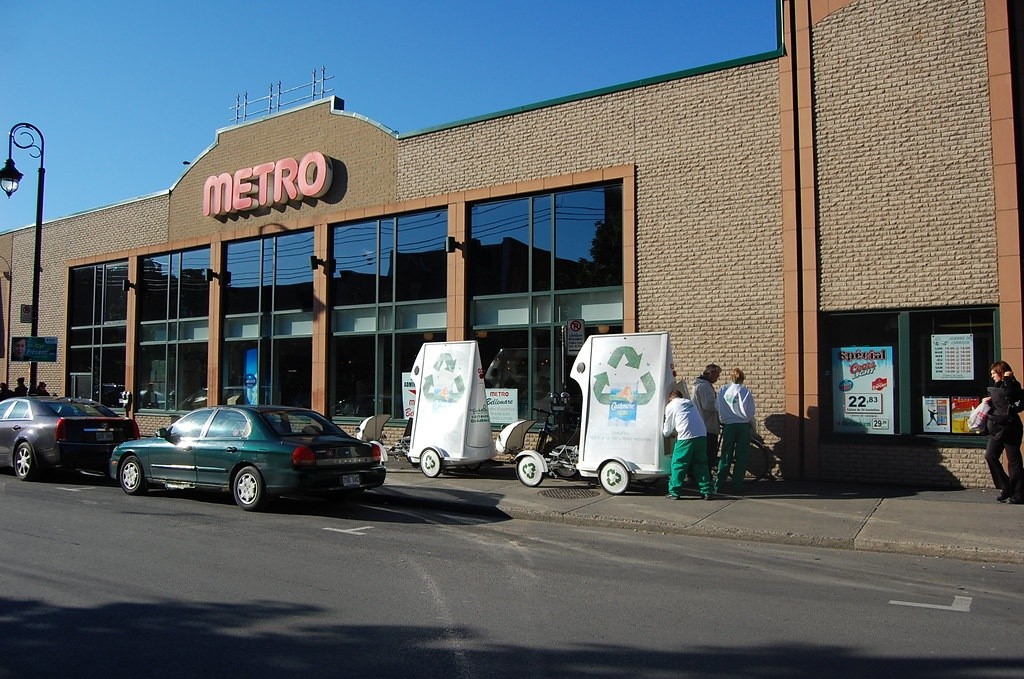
[
  {"x1": 968, "y1": 398, "x2": 991, "y2": 430},
  {"x1": 987, "y1": 414, "x2": 1013, "y2": 439}
]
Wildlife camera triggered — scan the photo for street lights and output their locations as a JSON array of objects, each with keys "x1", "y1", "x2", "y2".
[{"x1": 0, "y1": 121, "x2": 46, "y2": 394}]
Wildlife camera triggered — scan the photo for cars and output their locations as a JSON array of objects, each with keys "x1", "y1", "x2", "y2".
[
  {"x1": 94, "y1": 383, "x2": 171, "y2": 410},
  {"x1": 179, "y1": 384, "x2": 282, "y2": 409},
  {"x1": 0, "y1": 395, "x2": 141, "y2": 481},
  {"x1": 109, "y1": 405, "x2": 387, "y2": 511}
]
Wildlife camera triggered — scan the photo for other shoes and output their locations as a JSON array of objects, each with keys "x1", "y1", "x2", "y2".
[
  {"x1": 1007, "y1": 498, "x2": 1024, "y2": 503},
  {"x1": 701, "y1": 494, "x2": 711, "y2": 500},
  {"x1": 995, "y1": 493, "x2": 1010, "y2": 501},
  {"x1": 665, "y1": 493, "x2": 680, "y2": 499}
]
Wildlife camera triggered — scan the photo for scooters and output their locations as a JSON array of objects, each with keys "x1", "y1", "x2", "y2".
[
  {"x1": 355, "y1": 340, "x2": 498, "y2": 479},
  {"x1": 495, "y1": 331, "x2": 692, "y2": 496}
]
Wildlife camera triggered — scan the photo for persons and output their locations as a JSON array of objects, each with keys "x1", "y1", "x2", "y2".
[
  {"x1": 142, "y1": 381, "x2": 159, "y2": 408},
  {"x1": 12, "y1": 336, "x2": 31, "y2": 360},
  {"x1": 691, "y1": 363, "x2": 755, "y2": 495},
  {"x1": 663, "y1": 389, "x2": 716, "y2": 500},
  {"x1": 983, "y1": 359, "x2": 1023, "y2": 504},
  {"x1": 0, "y1": 376, "x2": 50, "y2": 401}
]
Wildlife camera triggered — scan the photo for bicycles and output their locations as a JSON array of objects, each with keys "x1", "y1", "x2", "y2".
[{"x1": 708, "y1": 422, "x2": 771, "y2": 485}]
